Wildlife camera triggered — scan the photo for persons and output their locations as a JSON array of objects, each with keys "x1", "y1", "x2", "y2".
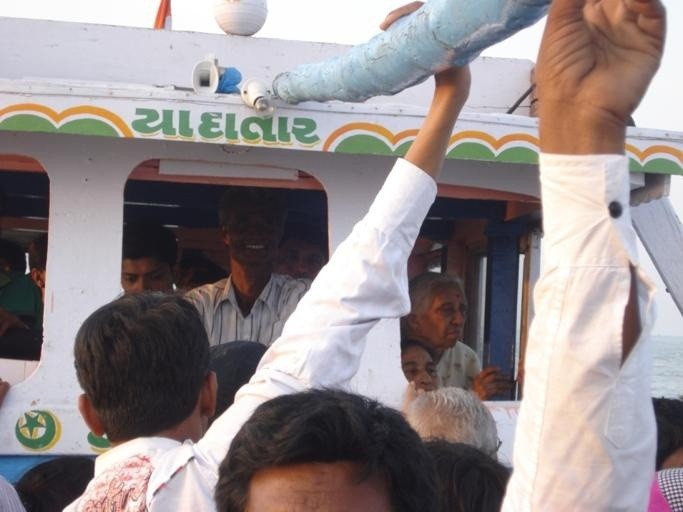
[
  {"x1": 401, "y1": 333, "x2": 441, "y2": 391},
  {"x1": 122, "y1": 219, "x2": 191, "y2": 296},
  {"x1": 180, "y1": 259, "x2": 229, "y2": 291},
  {"x1": 174, "y1": 248, "x2": 205, "y2": 286},
  {"x1": 211, "y1": 0, "x2": 671, "y2": 510},
  {"x1": 652, "y1": 396, "x2": 683, "y2": 469},
  {"x1": 56, "y1": 0, "x2": 475, "y2": 512},
  {"x1": 0, "y1": 238, "x2": 43, "y2": 329},
  {"x1": 1, "y1": 233, "x2": 48, "y2": 360},
  {"x1": 180, "y1": 187, "x2": 313, "y2": 350},
  {"x1": 402, "y1": 273, "x2": 516, "y2": 403},
  {"x1": 270, "y1": 224, "x2": 327, "y2": 278}
]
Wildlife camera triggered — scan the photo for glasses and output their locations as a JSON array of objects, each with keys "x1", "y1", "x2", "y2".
[{"x1": 240, "y1": 77, "x2": 276, "y2": 118}]
